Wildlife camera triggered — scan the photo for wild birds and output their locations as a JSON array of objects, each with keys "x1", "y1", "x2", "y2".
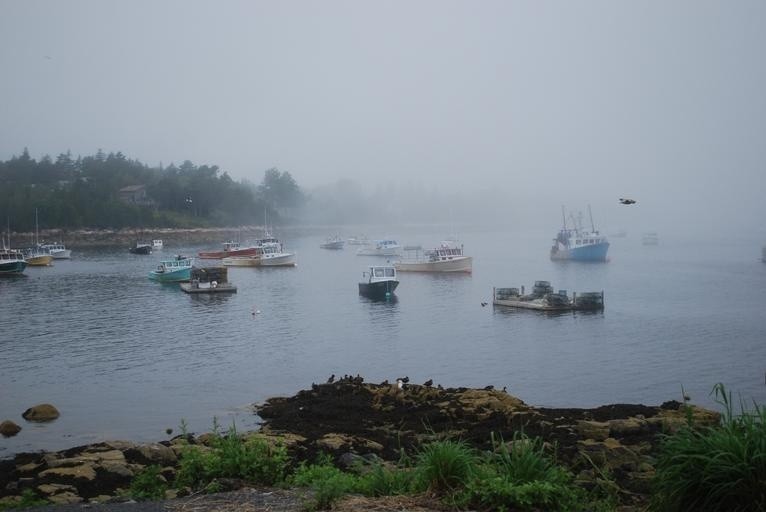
[
  {"x1": 424, "y1": 379, "x2": 433, "y2": 388},
  {"x1": 619, "y1": 197, "x2": 638, "y2": 204},
  {"x1": 328, "y1": 373, "x2": 364, "y2": 383},
  {"x1": 501, "y1": 386, "x2": 507, "y2": 393},
  {"x1": 395, "y1": 375, "x2": 410, "y2": 390}
]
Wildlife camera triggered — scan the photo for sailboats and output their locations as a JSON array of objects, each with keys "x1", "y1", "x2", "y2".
[
  {"x1": 2, "y1": 218, "x2": 29, "y2": 274},
  {"x1": 25, "y1": 208, "x2": 54, "y2": 266}
]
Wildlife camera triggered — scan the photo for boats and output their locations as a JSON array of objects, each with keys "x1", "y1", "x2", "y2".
[
  {"x1": 318, "y1": 231, "x2": 345, "y2": 251},
  {"x1": 357, "y1": 266, "x2": 399, "y2": 302},
  {"x1": 148, "y1": 254, "x2": 197, "y2": 285},
  {"x1": 179, "y1": 263, "x2": 238, "y2": 294},
  {"x1": 348, "y1": 224, "x2": 401, "y2": 257},
  {"x1": 391, "y1": 241, "x2": 474, "y2": 274},
  {"x1": 494, "y1": 280, "x2": 606, "y2": 315},
  {"x1": 128, "y1": 239, "x2": 153, "y2": 256},
  {"x1": 42, "y1": 242, "x2": 72, "y2": 259},
  {"x1": 194, "y1": 208, "x2": 296, "y2": 268},
  {"x1": 548, "y1": 203, "x2": 609, "y2": 262}
]
